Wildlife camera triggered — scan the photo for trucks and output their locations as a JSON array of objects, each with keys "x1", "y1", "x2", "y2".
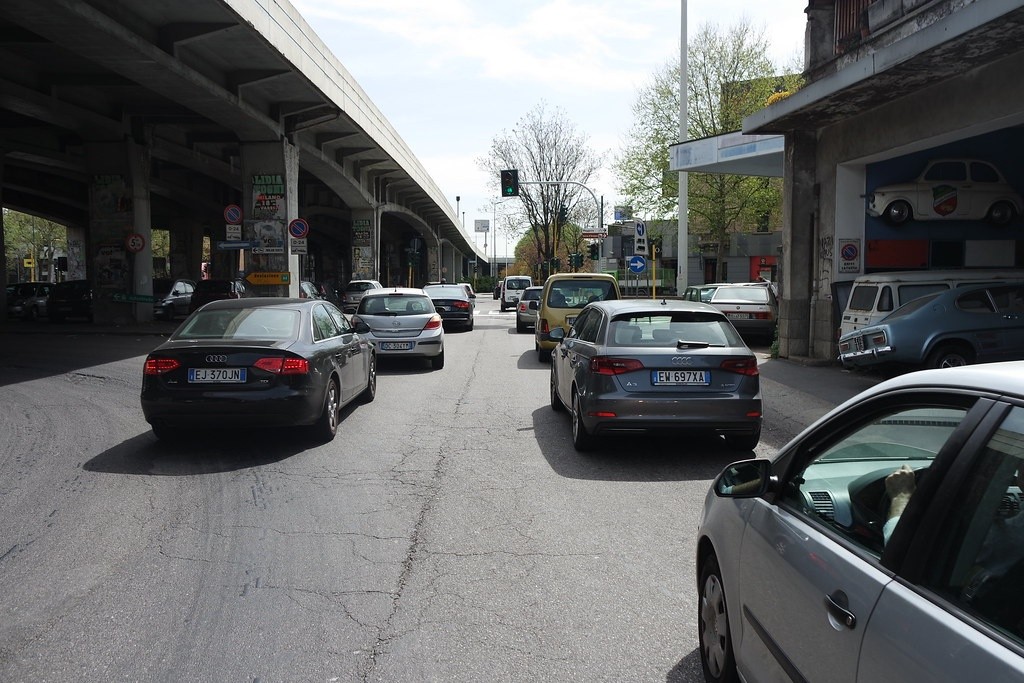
[{"x1": 582, "y1": 268, "x2": 676, "y2": 296}]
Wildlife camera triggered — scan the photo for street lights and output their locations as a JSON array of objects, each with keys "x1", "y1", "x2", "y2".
[
  {"x1": 493, "y1": 201, "x2": 502, "y2": 279},
  {"x1": 456, "y1": 196, "x2": 460, "y2": 217},
  {"x1": 568, "y1": 255, "x2": 573, "y2": 267},
  {"x1": 463, "y1": 211, "x2": 465, "y2": 228}
]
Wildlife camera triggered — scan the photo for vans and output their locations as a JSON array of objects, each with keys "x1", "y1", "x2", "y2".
[
  {"x1": 837, "y1": 268, "x2": 1024, "y2": 338},
  {"x1": 534, "y1": 271, "x2": 623, "y2": 362}
]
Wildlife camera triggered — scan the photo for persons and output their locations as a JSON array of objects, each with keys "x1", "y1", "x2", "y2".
[
  {"x1": 376, "y1": 298, "x2": 389, "y2": 311},
  {"x1": 882, "y1": 464, "x2": 917, "y2": 547},
  {"x1": 552, "y1": 289, "x2": 560, "y2": 302}
]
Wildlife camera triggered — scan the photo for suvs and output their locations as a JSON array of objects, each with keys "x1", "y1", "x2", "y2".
[
  {"x1": 493, "y1": 281, "x2": 503, "y2": 300},
  {"x1": 500, "y1": 276, "x2": 533, "y2": 312}
]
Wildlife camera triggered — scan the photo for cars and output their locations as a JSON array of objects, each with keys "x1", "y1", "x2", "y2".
[
  {"x1": 342, "y1": 280, "x2": 382, "y2": 314},
  {"x1": 355, "y1": 285, "x2": 445, "y2": 371},
  {"x1": 866, "y1": 156, "x2": 1024, "y2": 228},
  {"x1": 696, "y1": 360, "x2": 1024, "y2": 683},
  {"x1": 47, "y1": 280, "x2": 92, "y2": 321},
  {"x1": 710, "y1": 285, "x2": 778, "y2": 342},
  {"x1": 515, "y1": 285, "x2": 564, "y2": 333},
  {"x1": 682, "y1": 285, "x2": 716, "y2": 304},
  {"x1": 837, "y1": 282, "x2": 1024, "y2": 370},
  {"x1": 424, "y1": 278, "x2": 474, "y2": 333},
  {"x1": 190, "y1": 277, "x2": 245, "y2": 315},
  {"x1": 5, "y1": 282, "x2": 51, "y2": 320},
  {"x1": 457, "y1": 282, "x2": 477, "y2": 309},
  {"x1": 153, "y1": 277, "x2": 197, "y2": 322},
  {"x1": 299, "y1": 280, "x2": 323, "y2": 300},
  {"x1": 549, "y1": 299, "x2": 764, "y2": 453},
  {"x1": 140, "y1": 296, "x2": 376, "y2": 442}
]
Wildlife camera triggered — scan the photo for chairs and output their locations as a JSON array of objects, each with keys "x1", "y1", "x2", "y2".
[
  {"x1": 588, "y1": 296, "x2": 600, "y2": 305},
  {"x1": 530, "y1": 293, "x2": 537, "y2": 299},
  {"x1": 652, "y1": 329, "x2": 675, "y2": 340},
  {"x1": 369, "y1": 300, "x2": 384, "y2": 312},
  {"x1": 965, "y1": 499, "x2": 1024, "y2": 639},
  {"x1": 619, "y1": 325, "x2": 642, "y2": 343},
  {"x1": 406, "y1": 301, "x2": 422, "y2": 311},
  {"x1": 550, "y1": 291, "x2": 568, "y2": 308}
]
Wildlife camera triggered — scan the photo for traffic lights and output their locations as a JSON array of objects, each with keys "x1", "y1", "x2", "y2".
[
  {"x1": 572, "y1": 254, "x2": 577, "y2": 267},
  {"x1": 544, "y1": 259, "x2": 548, "y2": 270},
  {"x1": 578, "y1": 254, "x2": 584, "y2": 267},
  {"x1": 408, "y1": 253, "x2": 416, "y2": 266},
  {"x1": 501, "y1": 169, "x2": 519, "y2": 196},
  {"x1": 474, "y1": 266, "x2": 477, "y2": 273},
  {"x1": 586, "y1": 243, "x2": 599, "y2": 260},
  {"x1": 534, "y1": 264, "x2": 538, "y2": 273},
  {"x1": 204, "y1": 266, "x2": 207, "y2": 273}
]
